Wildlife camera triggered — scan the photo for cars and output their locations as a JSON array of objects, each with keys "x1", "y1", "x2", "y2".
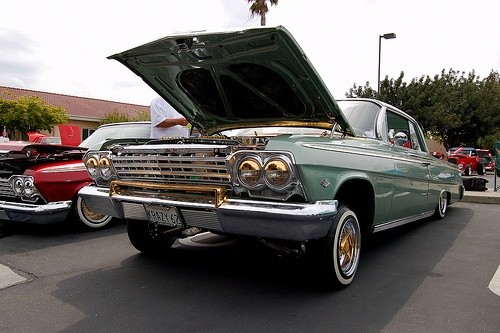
[
  {"x1": 78, "y1": 25, "x2": 465, "y2": 289},
  {"x1": 448, "y1": 147, "x2": 496, "y2": 175},
  {"x1": 0, "y1": 121, "x2": 151, "y2": 230}
]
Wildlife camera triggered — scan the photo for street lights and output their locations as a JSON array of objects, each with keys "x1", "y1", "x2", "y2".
[{"x1": 377, "y1": 33, "x2": 396, "y2": 98}]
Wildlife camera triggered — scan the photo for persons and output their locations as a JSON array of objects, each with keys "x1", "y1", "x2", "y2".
[{"x1": 150, "y1": 93, "x2": 189, "y2": 139}]
